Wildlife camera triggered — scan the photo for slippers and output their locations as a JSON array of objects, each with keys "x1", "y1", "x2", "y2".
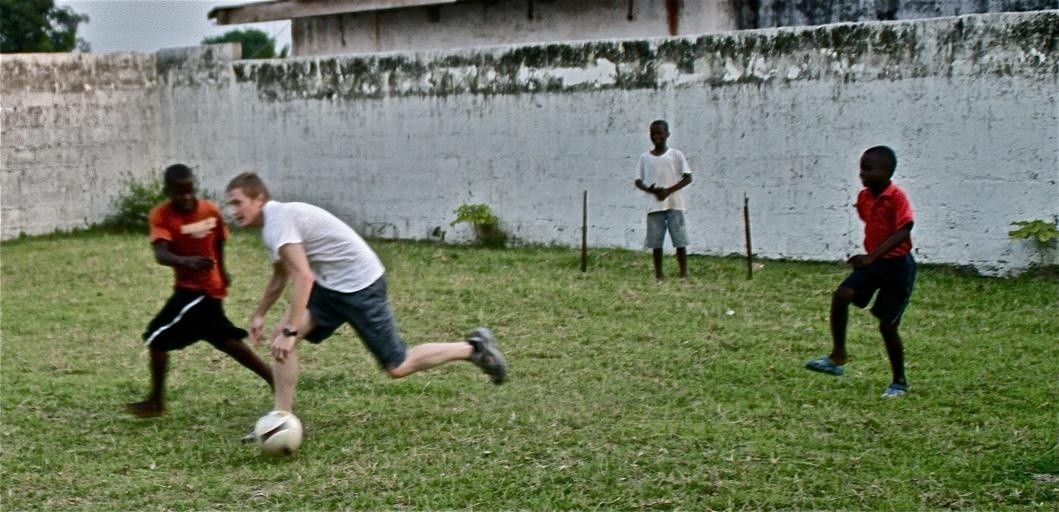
[
  {"x1": 880, "y1": 383, "x2": 906, "y2": 399},
  {"x1": 805, "y1": 357, "x2": 844, "y2": 376}
]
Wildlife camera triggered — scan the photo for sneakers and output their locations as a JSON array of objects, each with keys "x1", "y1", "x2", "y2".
[{"x1": 465, "y1": 327, "x2": 508, "y2": 385}]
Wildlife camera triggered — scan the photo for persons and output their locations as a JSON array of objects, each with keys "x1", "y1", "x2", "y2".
[
  {"x1": 634, "y1": 119, "x2": 692, "y2": 278},
  {"x1": 803, "y1": 146, "x2": 914, "y2": 398},
  {"x1": 226, "y1": 172, "x2": 507, "y2": 443},
  {"x1": 125, "y1": 164, "x2": 278, "y2": 416}
]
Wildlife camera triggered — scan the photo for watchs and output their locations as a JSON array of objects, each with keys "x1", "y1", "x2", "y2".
[{"x1": 279, "y1": 326, "x2": 298, "y2": 333}]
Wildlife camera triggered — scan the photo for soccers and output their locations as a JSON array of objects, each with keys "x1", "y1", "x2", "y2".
[{"x1": 255, "y1": 410, "x2": 302, "y2": 457}]
